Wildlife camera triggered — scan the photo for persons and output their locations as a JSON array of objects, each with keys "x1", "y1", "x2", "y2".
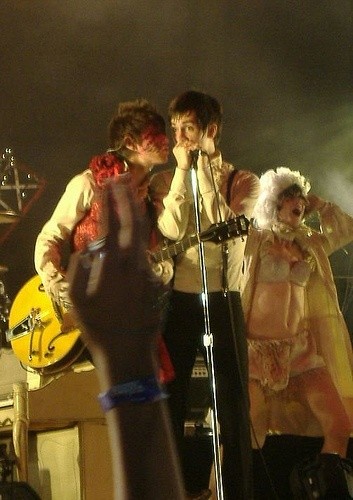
[
  {"x1": 158, "y1": 90, "x2": 262, "y2": 500},
  {"x1": 209, "y1": 165, "x2": 353, "y2": 500},
  {"x1": 31, "y1": 99, "x2": 184, "y2": 500}
]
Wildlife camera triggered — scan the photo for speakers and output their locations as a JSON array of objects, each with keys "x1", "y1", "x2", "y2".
[{"x1": 169, "y1": 361, "x2": 214, "y2": 497}]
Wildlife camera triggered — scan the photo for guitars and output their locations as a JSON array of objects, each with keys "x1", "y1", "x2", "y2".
[{"x1": 9, "y1": 215, "x2": 251, "y2": 375}]
